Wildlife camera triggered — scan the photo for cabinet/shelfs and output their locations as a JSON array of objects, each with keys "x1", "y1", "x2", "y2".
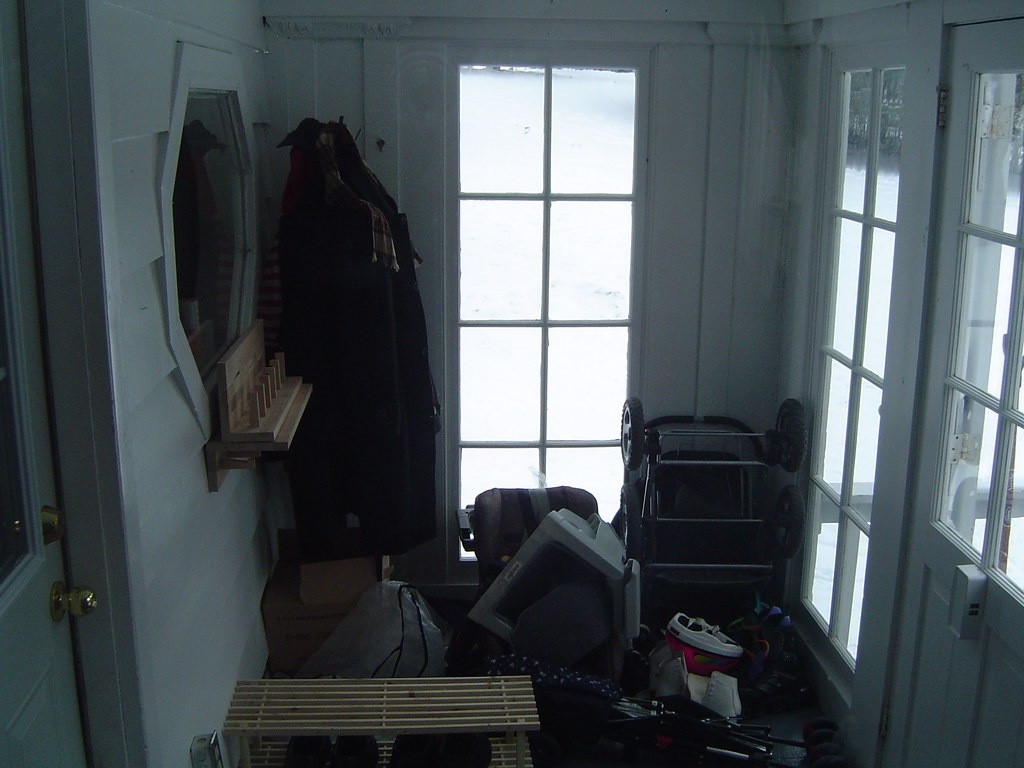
[{"x1": 222, "y1": 674, "x2": 539, "y2": 768}]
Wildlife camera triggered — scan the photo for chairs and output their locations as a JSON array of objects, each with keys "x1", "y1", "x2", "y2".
[{"x1": 473, "y1": 486, "x2": 625, "y2": 693}]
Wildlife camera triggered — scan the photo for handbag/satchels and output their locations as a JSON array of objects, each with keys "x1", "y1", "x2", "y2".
[{"x1": 299, "y1": 581, "x2": 446, "y2": 677}]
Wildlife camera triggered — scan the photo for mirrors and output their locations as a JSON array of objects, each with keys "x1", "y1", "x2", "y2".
[{"x1": 155, "y1": 41, "x2": 259, "y2": 441}]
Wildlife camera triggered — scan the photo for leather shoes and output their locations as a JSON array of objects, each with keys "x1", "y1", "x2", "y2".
[
  {"x1": 749, "y1": 671, "x2": 784, "y2": 696},
  {"x1": 751, "y1": 686, "x2": 812, "y2": 707}
]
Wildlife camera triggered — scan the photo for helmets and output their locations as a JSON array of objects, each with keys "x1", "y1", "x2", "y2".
[{"x1": 663, "y1": 613, "x2": 743, "y2": 677}]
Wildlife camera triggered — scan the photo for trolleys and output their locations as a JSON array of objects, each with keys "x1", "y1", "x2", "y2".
[{"x1": 621, "y1": 397, "x2": 810, "y2": 634}]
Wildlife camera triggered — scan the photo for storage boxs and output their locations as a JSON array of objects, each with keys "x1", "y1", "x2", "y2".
[{"x1": 260, "y1": 556, "x2": 378, "y2": 667}]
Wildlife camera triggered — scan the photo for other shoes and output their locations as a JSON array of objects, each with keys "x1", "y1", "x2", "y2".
[
  {"x1": 689, "y1": 671, "x2": 741, "y2": 718},
  {"x1": 390, "y1": 734, "x2": 438, "y2": 768},
  {"x1": 438, "y1": 733, "x2": 491, "y2": 768},
  {"x1": 283, "y1": 735, "x2": 330, "y2": 768},
  {"x1": 649, "y1": 639, "x2": 692, "y2": 700},
  {"x1": 330, "y1": 735, "x2": 378, "y2": 768}
]
